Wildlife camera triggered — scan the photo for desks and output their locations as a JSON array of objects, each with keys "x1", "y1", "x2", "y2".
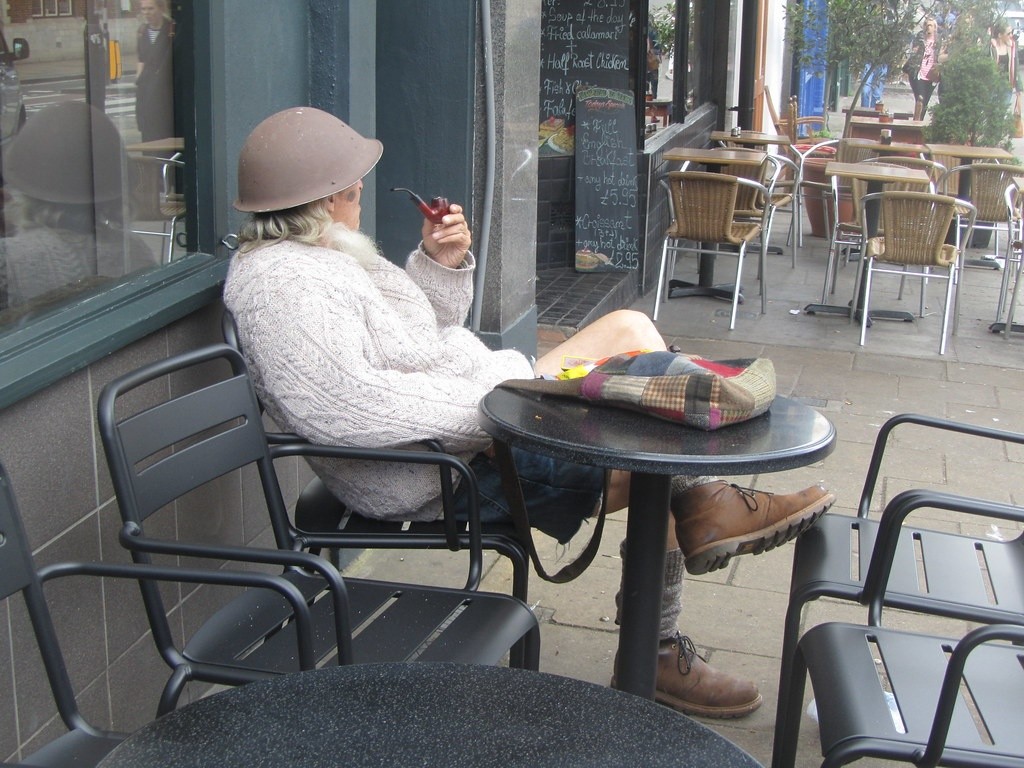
[
  {"x1": 850, "y1": 116, "x2": 925, "y2": 142},
  {"x1": 804, "y1": 161, "x2": 930, "y2": 329},
  {"x1": 841, "y1": 137, "x2": 930, "y2": 260},
  {"x1": 96, "y1": 662, "x2": 764, "y2": 768},
  {"x1": 842, "y1": 105, "x2": 914, "y2": 120},
  {"x1": 709, "y1": 130, "x2": 790, "y2": 149},
  {"x1": 927, "y1": 142, "x2": 1012, "y2": 246},
  {"x1": 662, "y1": 146, "x2": 769, "y2": 306},
  {"x1": 477, "y1": 388, "x2": 837, "y2": 699}
]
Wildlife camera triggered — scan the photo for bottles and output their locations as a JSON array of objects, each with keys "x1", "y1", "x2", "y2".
[{"x1": 731, "y1": 126, "x2": 741, "y2": 136}]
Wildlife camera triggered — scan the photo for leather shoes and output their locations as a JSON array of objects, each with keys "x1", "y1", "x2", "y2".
[
  {"x1": 669, "y1": 480, "x2": 837, "y2": 575},
  {"x1": 610, "y1": 630, "x2": 764, "y2": 718}
]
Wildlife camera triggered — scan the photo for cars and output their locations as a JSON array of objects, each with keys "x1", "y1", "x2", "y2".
[{"x1": 0, "y1": 24, "x2": 31, "y2": 143}]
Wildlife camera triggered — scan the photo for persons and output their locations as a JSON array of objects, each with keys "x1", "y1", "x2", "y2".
[
  {"x1": 0, "y1": 101, "x2": 153, "y2": 320},
  {"x1": 648, "y1": 2, "x2": 694, "y2": 123},
  {"x1": 939, "y1": 16, "x2": 978, "y2": 105},
  {"x1": 862, "y1": 59, "x2": 891, "y2": 108},
  {"x1": 909, "y1": 19, "x2": 943, "y2": 122},
  {"x1": 989, "y1": 22, "x2": 1024, "y2": 150},
  {"x1": 222, "y1": 106, "x2": 839, "y2": 719},
  {"x1": 136, "y1": 0, "x2": 174, "y2": 185}
]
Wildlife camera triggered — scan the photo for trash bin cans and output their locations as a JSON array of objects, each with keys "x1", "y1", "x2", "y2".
[{"x1": 108, "y1": 40, "x2": 122, "y2": 80}]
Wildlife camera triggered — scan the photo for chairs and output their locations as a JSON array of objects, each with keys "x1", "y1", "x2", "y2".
[
  {"x1": 771, "y1": 412, "x2": 1024, "y2": 768},
  {"x1": 1, "y1": 311, "x2": 540, "y2": 768},
  {"x1": 654, "y1": 86, "x2": 1024, "y2": 355}
]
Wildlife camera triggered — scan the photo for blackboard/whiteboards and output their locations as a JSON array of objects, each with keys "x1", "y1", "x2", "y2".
[
  {"x1": 628, "y1": 0, "x2": 642, "y2": 135},
  {"x1": 574, "y1": 85, "x2": 640, "y2": 273},
  {"x1": 538, "y1": 0, "x2": 631, "y2": 160}
]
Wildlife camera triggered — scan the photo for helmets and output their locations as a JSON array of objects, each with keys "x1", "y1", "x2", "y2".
[
  {"x1": 2, "y1": 102, "x2": 129, "y2": 203},
  {"x1": 231, "y1": 106, "x2": 383, "y2": 212}
]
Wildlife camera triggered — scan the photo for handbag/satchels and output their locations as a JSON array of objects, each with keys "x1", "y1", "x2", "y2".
[
  {"x1": 901, "y1": 48, "x2": 923, "y2": 76},
  {"x1": 926, "y1": 48, "x2": 938, "y2": 80},
  {"x1": 1010, "y1": 93, "x2": 1024, "y2": 138},
  {"x1": 494, "y1": 346, "x2": 776, "y2": 583}
]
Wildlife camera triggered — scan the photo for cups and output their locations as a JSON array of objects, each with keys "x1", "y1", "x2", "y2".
[{"x1": 881, "y1": 129, "x2": 892, "y2": 145}]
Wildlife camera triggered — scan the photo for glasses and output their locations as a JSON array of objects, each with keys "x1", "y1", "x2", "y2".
[{"x1": 1007, "y1": 29, "x2": 1014, "y2": 35}]
